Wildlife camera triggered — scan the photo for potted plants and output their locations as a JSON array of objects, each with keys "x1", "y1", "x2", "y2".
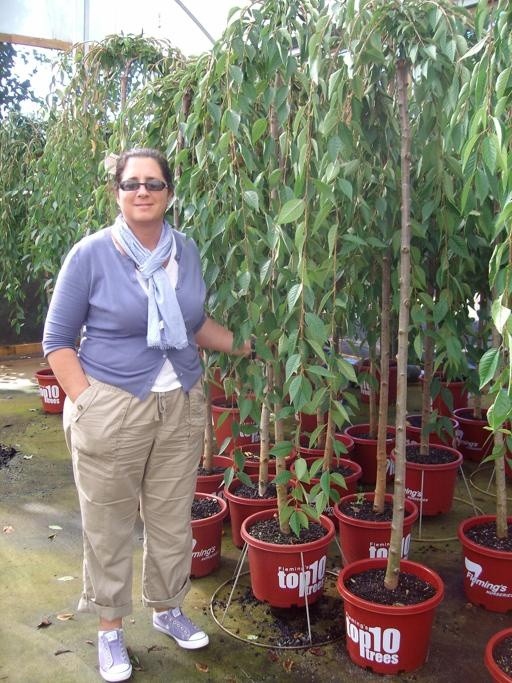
[
  {"x1": 159, "y1": 1, "x2": 512, "y2": 678},
  {"x1": 29, "y1": 71, "x2": 91, "y2": 424}
]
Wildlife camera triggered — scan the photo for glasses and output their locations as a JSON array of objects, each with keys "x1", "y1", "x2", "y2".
[{"x1": 120, "y1": 179, "x2": 169, "y2": 191}]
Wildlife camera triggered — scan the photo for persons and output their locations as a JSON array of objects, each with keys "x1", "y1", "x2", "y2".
[{"x1": 42, "y1": 149, "x2": 279, "y2": 683}]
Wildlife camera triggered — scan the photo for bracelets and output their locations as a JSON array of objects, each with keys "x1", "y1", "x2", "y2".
[{"x1": 251, "y1": 336, "x2": 257, "y2": 360}]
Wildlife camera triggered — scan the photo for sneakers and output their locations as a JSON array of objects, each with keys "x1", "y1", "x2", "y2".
[
  {"x1": 153, "y1": 608, "x2": 209, "y2": 649},
  {"x1": 98, "y1": 629, "x2": 132, "y2": 682}
]
